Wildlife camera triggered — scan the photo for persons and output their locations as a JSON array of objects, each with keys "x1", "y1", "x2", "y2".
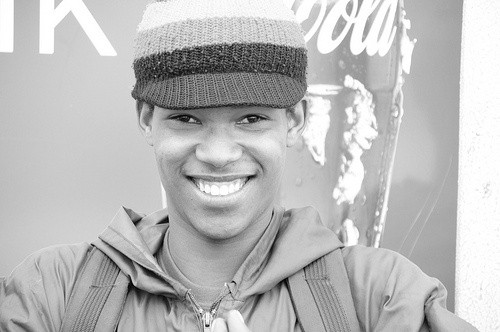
[{"x1": 1, "y1": 0, "x2": 478, "y2": 332}]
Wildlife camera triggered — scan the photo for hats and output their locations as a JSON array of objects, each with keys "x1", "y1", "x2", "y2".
[{"x1": 131, "y1": 0, "x2": 308, "y2": 110}]
